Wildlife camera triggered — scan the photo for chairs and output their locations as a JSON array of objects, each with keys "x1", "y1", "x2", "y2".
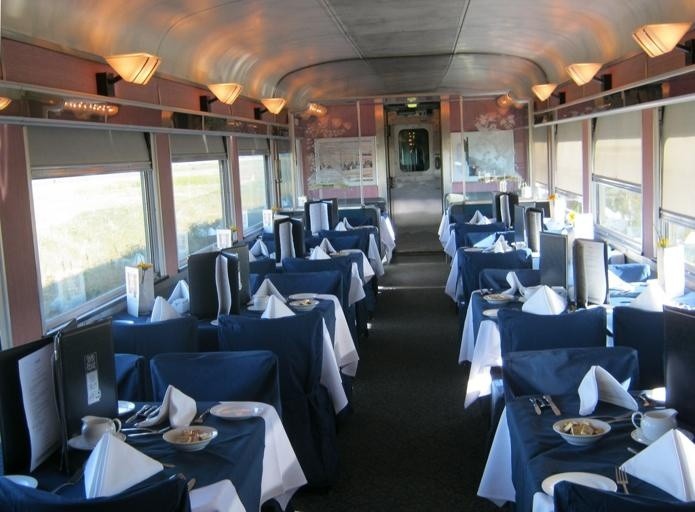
[
  {"x1": 0, "y1": 194, "x2": 392, "y2": 512},
  {"x1": 442, "y1": 186, "x2": 695, "y2": 512}
]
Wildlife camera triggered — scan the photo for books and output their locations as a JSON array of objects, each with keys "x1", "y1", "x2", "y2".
[
  {"x1": 515, "y1": 201, "x2": 550, "y2": 252},
  {"x1": 187, "y1": 245, "x2": 251, "y2": 319},
  {"x1": 305, "y1": 197, "x2": 338, "y2": 233},
  {"x1": 0, "y1": 316, "x2": 119, "y2": 477},
  {"x1": 491, "y1": 191, "x2": 518, "y2": 228},
  {"x1": 274, "y1": 215, "x2": 305, "y2": 263},
  {"x1": 540, "y1": 231, "x2": 610, "y2": 309}
]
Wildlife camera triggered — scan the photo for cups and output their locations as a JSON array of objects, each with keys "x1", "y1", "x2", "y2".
[
  {"x1": 253, "y1": 294, "x2": 266, "y2": 307},
  {"x1": 631, "y1": 408, "x2": 678, "y2": 442},
  {"x1": 80, "y1": 416, "x2": 122, "y2": 443}
]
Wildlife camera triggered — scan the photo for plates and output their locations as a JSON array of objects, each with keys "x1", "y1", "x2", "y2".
[
  {"x1": 117, "y1": 399, "x2": 135, "y2": 416},
  {"x1": 246, "y1": 306, "x2": 265, "y2": 312},
  {"x1": 481, "y1": 308, "x2": 498, "y2": 318},
  {"x1": 329, "y1": 252, "x2": 350, "y2": 256},
  {"x1": 646, "y1": 388, "x2": 666, "y2": 401},
  {"x1": 210, "y1": 320, "x2": 219, "y2": 327},
  {"x1": 210, "y1": 404, "x2": 265, "y2": 421},
  {"x1": 288, "y1": 292, "x2": 317, "y2": 300},
  {"x1": 541, "y1": 471, "x2": 618, "y2": 498},
  {"x1": 627, "y1": 426, "x2": 694, "y2": 447},
  {"x1": 4, "y1": 474, "x2": 39, "y2": 489},
  {"x1": 463, "y1": 248, "x2": 483, "y2": 253},
  {"x1": 511, "y1": 241, "x2": 525, "y2": 247},
  {"x1": 66, "y1": 433, "x2": 125, "y2": 451}
]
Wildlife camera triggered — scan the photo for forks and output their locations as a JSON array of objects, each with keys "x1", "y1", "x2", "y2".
[
  {"x1": 638, "y1": 395, "x2": 653, "y2": 407},
  {"x1": 194, "y1": 409, "x2": 211, "y2": 424},
  {"x1": 136, "y1": 405, "x2": 156, "y2": 418},
  {"x1": 586, "y1": 411, "x2": 631, "y2": 420},
  {"x1": 50, "y1": 465, "x2": 83, "y2": 494},
  {"x1": 614, "y1": 463, "x2": 631, "y2": 493},
  {"x1": 536, "y1": 396, "x2": 550, "y2": 407},
  {"x1": 120, "y1": 426, "x2": 170, "y2": 437}
]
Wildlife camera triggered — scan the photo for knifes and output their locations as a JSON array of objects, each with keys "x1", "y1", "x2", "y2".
[
  {"x1": 542, "y1": 392, "x2": 561, "y2": 418},
  {"x1": 604, "y1": 415, "x2": 641, "y2": 423},
  {"x1": 186, "y1": 478, "x2": 196, "y2": 491},
  {"x1": 529, "y1": 396, "x2": 542, "y2": 416},
  {"x1": 133, "y1": 406, "x2": 161, "y2": 425},
  {"x1": 124, "y1": 403, "x2": 151, "y2": 424}
]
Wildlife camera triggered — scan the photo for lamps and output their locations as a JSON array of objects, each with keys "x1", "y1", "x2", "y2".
[
  {"x1": 630, "y1": 20, "x2": 694, "y2": 63},
  {"x1": 304, "y1": 100, "x2": 328, "y2": 119},
  {"x1": 103, "y1": 51, "x2": 159, "y2": 87},
  {"x1": 531, "y1": 80, "x2": 558, "y2": 104},
  {"x1": 561, "y1": 59, "x2": 604, "y2": 89},
  {"x1": 261, "y1": 95, "x2": 287, "y2": 118},
  {"x1": 493, "y1": 94, "x2": 513, "y2": 109},
  {"x1": 205, "y1": 79, "x2": 242, "y2": 107}
]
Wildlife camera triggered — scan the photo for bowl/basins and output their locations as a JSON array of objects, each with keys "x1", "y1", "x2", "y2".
[
  {"x1": 289, "y1": 298, "x2": 319, "y2": 313},
  {"x1": 482, "y1": 295, "x2": 515, "y2": 304},
  {"x1": 160, "y1": 426, "x2": 219, "y2": 453},
  {"x1": 552, "y1": 416, "x2": 612, "y2": 448}
]
